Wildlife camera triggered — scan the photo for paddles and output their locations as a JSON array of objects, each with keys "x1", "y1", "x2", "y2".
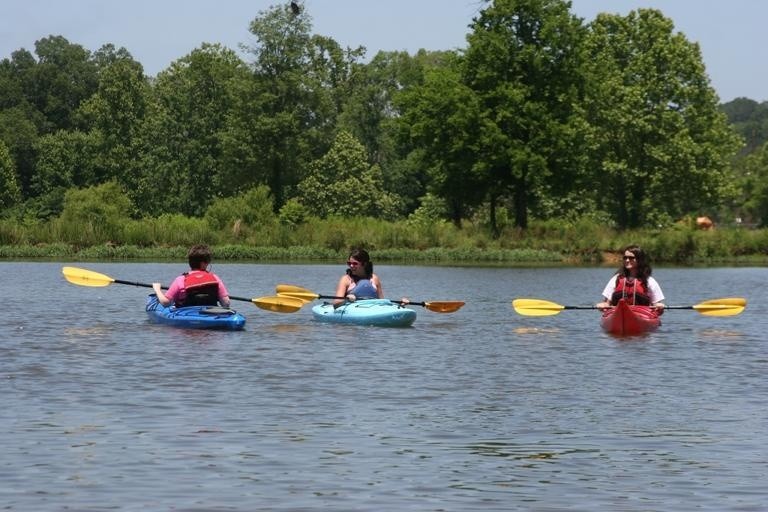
[
  {"x1": 512, "y1": 298, "x2": 746, "y2": 316},
  {"x1": 276, "y1": 285, "x2": 465, "y2": 313},
  {"x1": 62, "y1": 267, "x2": 303, "y2": 313}
]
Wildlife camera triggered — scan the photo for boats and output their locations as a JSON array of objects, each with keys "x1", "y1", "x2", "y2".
[
  {"x1": 310, "y1": 298, "x2": 416, "y2": 328},
  {"x1": 597, "y1": 299, "x2": 659, "y2": 337},
  {"x1": 143, "y1": 294, "x2": 245, "y2": 331}
]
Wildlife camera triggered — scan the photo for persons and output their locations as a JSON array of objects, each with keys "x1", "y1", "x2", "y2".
[
  {"x1": 333, "y1": 251, "x2": 412, "y2": 310},
  {"x1": 594, "y1": 246, "x2": 666, "y2": 316},
  {"x1": 152, "y1": 245, "x2": 232, "y2": 309}
]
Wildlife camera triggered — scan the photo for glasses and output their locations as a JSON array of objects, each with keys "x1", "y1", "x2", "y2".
[
  {"x1": 347, "y1": 262, "x2": 358, "y2": 265},
  {"x1": 623, "y1": 256, "x2": 636, "y2": 260}
]
